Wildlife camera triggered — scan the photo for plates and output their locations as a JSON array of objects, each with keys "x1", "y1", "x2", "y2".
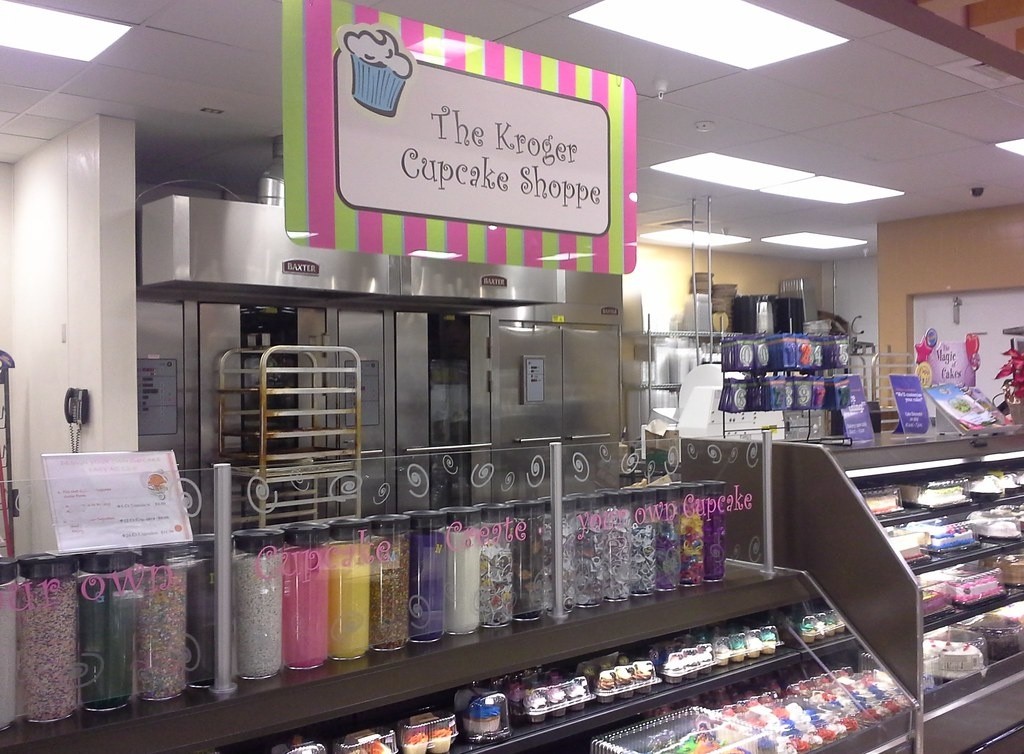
[
  {"x1": 969, "y1": 490, "x2": 1003, "y2": 503},
  {"x1": 1005, "y1": 485, "x2": 1022, "y2": 497}
]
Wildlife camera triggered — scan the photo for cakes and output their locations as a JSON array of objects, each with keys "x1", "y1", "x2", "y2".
[{"x1": 864, "y1": 466, "x2": 1024, "y2": 691}]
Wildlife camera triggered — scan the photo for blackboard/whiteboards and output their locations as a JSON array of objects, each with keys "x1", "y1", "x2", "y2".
[{"x1": 138, "y1": 359, "x2": 177, "y2": 435}]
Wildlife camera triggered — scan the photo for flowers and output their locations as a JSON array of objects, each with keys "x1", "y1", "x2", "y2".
[{"x1": 993, "y1": 338, "x2": 1024, "y2": 402}]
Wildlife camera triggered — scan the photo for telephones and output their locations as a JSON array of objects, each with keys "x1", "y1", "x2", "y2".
[{"x1": 64, "y1": 387, "x2": 89, "y2": 424}]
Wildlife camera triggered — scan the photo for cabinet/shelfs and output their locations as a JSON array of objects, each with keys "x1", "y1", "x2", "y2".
[
  {"x1": 216, "y1": 345, "x2": 363, "y2": 529},
  {"x1": 0, "y1": 434, "x2": 1024, "y2": 754},
  {"x1": 623, "y1": 294, "x2": 875, "y2": 435}
]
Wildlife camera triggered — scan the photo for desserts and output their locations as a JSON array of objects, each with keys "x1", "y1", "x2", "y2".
[{"x1": 274, "y1": 605, "x2": 907, "y2": 754}]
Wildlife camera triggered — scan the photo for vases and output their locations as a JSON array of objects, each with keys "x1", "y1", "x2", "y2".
[{"x1": 1004, "y1": 398, "x2": 1024, "y2": 424}]
